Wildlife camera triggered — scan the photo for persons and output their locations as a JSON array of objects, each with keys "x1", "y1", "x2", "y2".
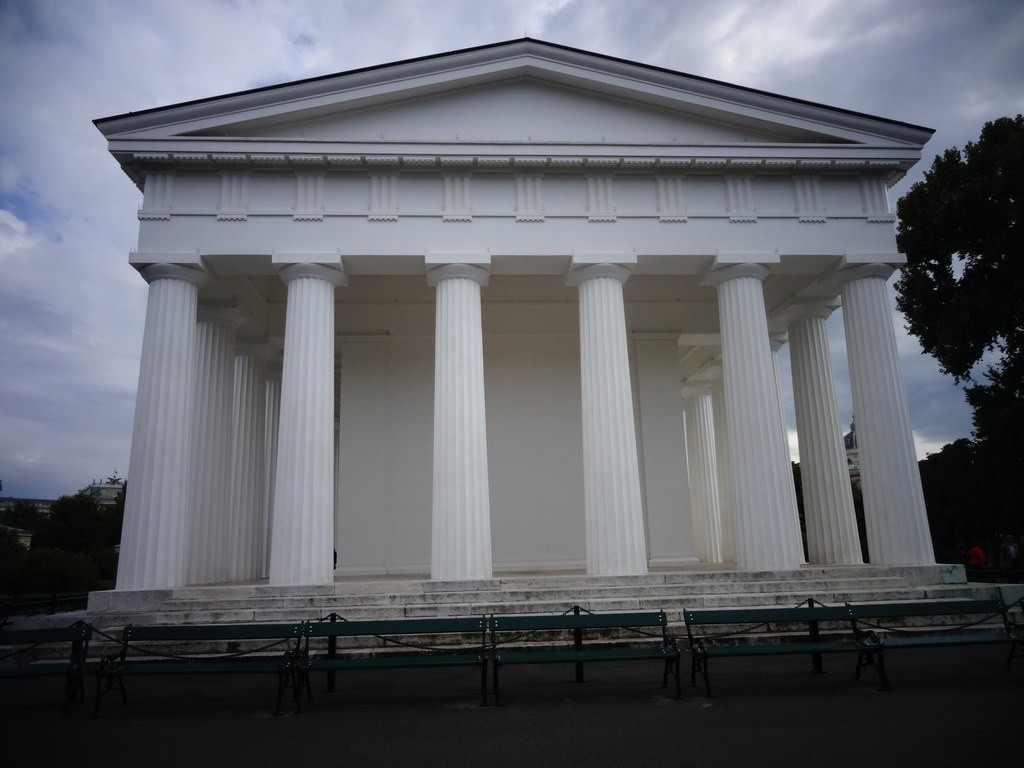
[
  {"x1": 968, "y1": 541, "x2": 986, "y2": 583},
  {"x1": 999, "y1": 534, "x2": 1022, "y2": 583}
]
